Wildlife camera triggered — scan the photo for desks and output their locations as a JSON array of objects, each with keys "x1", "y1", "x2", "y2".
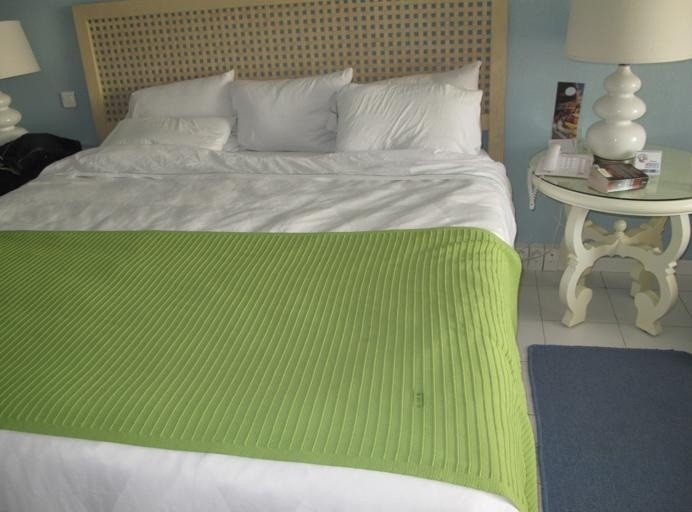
[{"x1": 528, "y1": 143, "x2": 692, "y2": 337}]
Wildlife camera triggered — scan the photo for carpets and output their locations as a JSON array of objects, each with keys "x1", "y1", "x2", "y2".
[{"x1": 527, "y1": 344, "x2": 691, "y2": 511}]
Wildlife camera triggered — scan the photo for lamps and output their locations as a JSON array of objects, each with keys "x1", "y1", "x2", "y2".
[
  {"x1": 1, "y1": 16, "x2": 41, "y2": 146},
  {"x1": 563, "y1": 1, "x2": 692, "y2": 161}
]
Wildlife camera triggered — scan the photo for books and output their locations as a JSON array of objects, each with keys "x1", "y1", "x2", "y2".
[{"x1": 586, "y1": 163, "x2": 648, "y2": 194}]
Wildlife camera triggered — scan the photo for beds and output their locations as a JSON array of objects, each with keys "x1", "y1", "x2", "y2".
[{"x1": 0, "y1": 0, "x2": 544, "y2": 512}]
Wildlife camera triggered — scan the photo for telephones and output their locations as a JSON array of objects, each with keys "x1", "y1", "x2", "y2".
[{"x1": 534, "y1": 143, "x2": 594, "y2": 180}]
